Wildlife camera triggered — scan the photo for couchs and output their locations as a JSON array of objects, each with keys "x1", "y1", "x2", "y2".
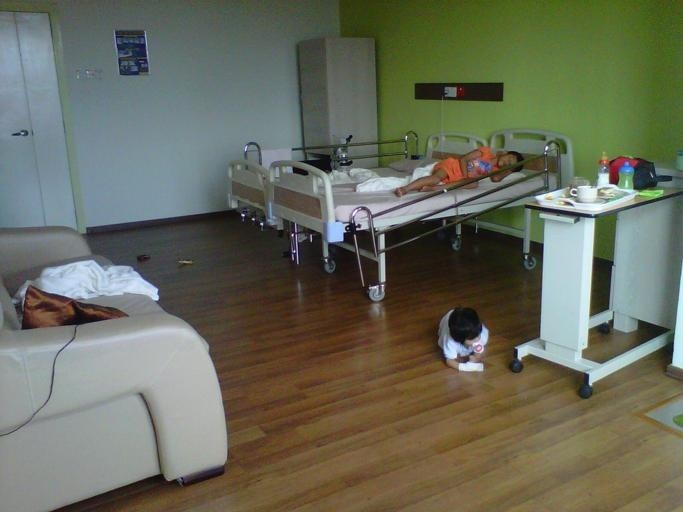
[{"x1": 0, "y1": 225, "x2": 228, "y2": 512}]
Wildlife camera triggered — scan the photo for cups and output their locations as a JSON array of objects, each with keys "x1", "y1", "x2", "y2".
[
  {"x1": 572, "y1": 177, "x2": 589, "y2": 188},
  {"x1": 570, "y1": 186, "x2": 597, "y2": 203},
  {"x1": 676, "y1": 152, "x2": 683, "y2": 171}
]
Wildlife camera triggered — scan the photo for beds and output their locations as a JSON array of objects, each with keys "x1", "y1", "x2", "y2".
[
  {"x1": 228, "y1": 133, "x2": 488, "y2": 256},
  {"x1": 265, "y1": 127, "x2": 572, "y2": 300}
]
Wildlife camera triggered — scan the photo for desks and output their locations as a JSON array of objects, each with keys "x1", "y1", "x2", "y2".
[{"x1": 508, "y1": 161, "x2": 683, "y2": 399}]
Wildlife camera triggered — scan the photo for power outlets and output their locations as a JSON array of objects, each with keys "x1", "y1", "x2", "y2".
[{"x1": 444, "y1": 86, "x2": 457, "y2": 97}]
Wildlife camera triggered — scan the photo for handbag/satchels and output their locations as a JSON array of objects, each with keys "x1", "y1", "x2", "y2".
[{"x1": 609, "y1": 155, "x2": 672, "y2": 190}]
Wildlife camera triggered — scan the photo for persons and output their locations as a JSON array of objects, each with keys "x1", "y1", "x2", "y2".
[
  {"x1": 395, "y1": 146, "x2": 525, "y2": 198},
  {"x1": 436, "y1": 305, "x2": 490, "y2": 373}
]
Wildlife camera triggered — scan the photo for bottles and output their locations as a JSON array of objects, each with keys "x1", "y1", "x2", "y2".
[
  {"x1": 596, "y1": 152, "x2": 610, "y2": 188},
  {"x1": 618, "y1": 159, "x2": 634, "y2": 191}
]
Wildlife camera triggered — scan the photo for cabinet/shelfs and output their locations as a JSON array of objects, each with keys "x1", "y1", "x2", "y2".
[{"x1": 298, "y1": 36, "x2": 378, "y2": 171}]
